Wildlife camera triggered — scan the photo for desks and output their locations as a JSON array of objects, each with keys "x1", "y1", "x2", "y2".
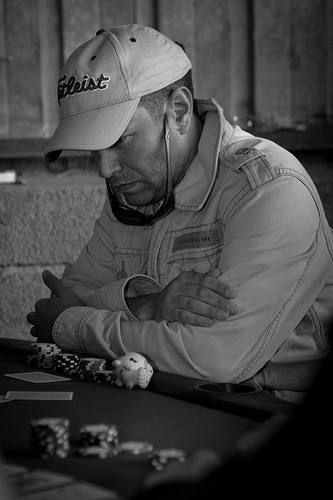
[{"x1": 0, "y1": 335, "x2": 321, "y2": 500}]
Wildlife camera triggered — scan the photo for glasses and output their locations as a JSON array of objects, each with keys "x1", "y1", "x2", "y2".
[{"x1": 106, "y1": 113, "x2": 175, "y2": 228}]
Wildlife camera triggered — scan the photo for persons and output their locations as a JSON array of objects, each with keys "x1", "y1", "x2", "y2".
[{"x1": 27, "y1": 24, "x2": 333, "y2": 407}]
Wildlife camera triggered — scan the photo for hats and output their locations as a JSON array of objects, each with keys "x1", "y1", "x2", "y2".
[{"x1": 42, "y1": 25, "x2": 194, "y2": 165}]
